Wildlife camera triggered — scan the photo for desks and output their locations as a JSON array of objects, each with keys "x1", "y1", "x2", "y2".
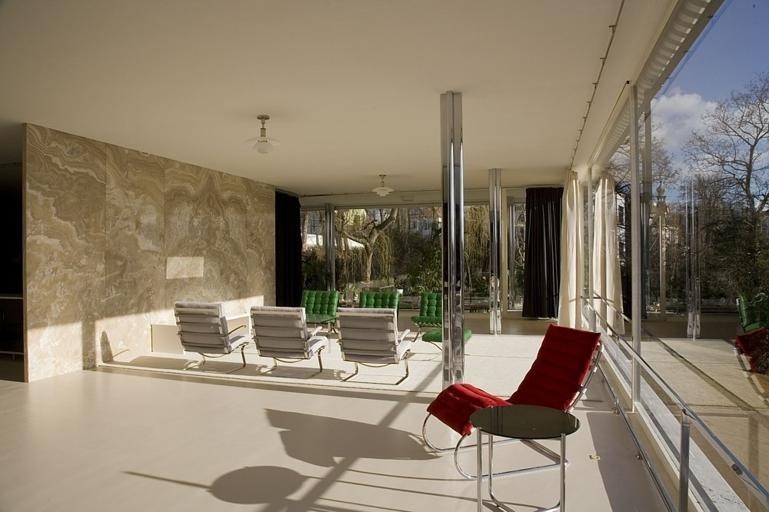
[{"x1": 151, "y1": 313, "x2": 249, "y2": 355}]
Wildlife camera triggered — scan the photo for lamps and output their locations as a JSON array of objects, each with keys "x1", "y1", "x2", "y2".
[
  {"x1": 246, "y1": 114, "x2": 281, "y2": 154},
  {"x1": 369, "y1": 174, "x2": 395, "y2": 197}
]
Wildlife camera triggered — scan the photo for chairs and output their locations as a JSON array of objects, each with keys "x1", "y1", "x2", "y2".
[
  {"x1": 422, "y1": 324, "x2": 603, "y2": 482},
  {"x1": 174, "y1": 288, "x2": 473, "y2": 386},
  {"x1": 733, "y1": 325, "x2": 769, "y2": 406}
]
